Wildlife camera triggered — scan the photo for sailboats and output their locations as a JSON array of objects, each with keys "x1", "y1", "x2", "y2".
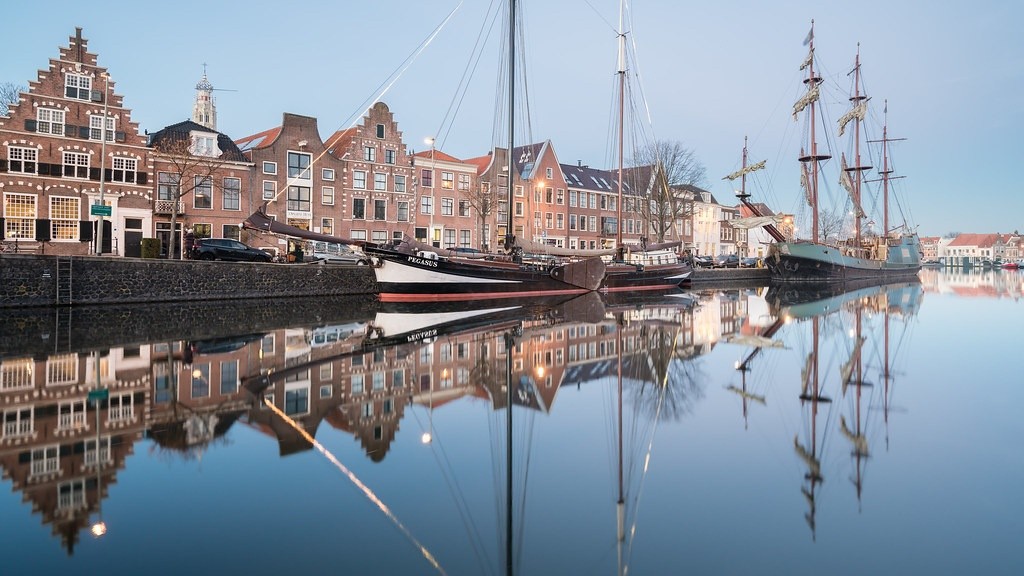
[
  {"x1": 239, "y1": 10, "x2": 696, "y2": 312},
  {"x1": 731, "y1": 14, "x2": 925, "y2": 282},
  {"x1": 739, "y1": 274, "x2": 927, "y2": 542},
  {"x1": 242, "y1": 292, "x2": 702, "y2": 576}
]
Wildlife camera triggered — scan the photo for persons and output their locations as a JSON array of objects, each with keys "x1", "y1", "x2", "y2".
[{"x1": 186, "y1": 228, "x2": 195, "y2": 258}]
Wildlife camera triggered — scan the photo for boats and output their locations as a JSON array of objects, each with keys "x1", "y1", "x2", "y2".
[{"x1": 981, "y1": 259, "x2": 1024, "y2": 272}]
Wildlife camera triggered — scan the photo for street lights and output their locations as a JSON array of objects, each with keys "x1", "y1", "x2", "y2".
[
  {"x1": 424, "y1": 137, "x2": 435, "y2": 247},
  {"x1": 96, "y1": 72, "x2": 110, "y2": 252}
]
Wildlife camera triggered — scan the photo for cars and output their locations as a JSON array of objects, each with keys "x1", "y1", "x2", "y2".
[
  {"x1": 191, "y1": 236, "x2": 274, "y2": 262},
  {"x1": 714, "y1": 254, "x2": 740, "y2": 269},
  {"x1": 446, "y1": 247, "x2": 479, "y2": 254},
  {"x1": 679, "y1": 254, "x2": 715, "y2": 269},
  {"x1": 302, "y1": 240, "x2": 371, "y2": 266},
  {"x1": 740, "y1": 256, "x2": 761, "y2": 268}
]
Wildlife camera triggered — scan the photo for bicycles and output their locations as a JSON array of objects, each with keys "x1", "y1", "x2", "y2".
[{"x1": 3, "y1": 242, "x2": 22, "y2": 253}]
